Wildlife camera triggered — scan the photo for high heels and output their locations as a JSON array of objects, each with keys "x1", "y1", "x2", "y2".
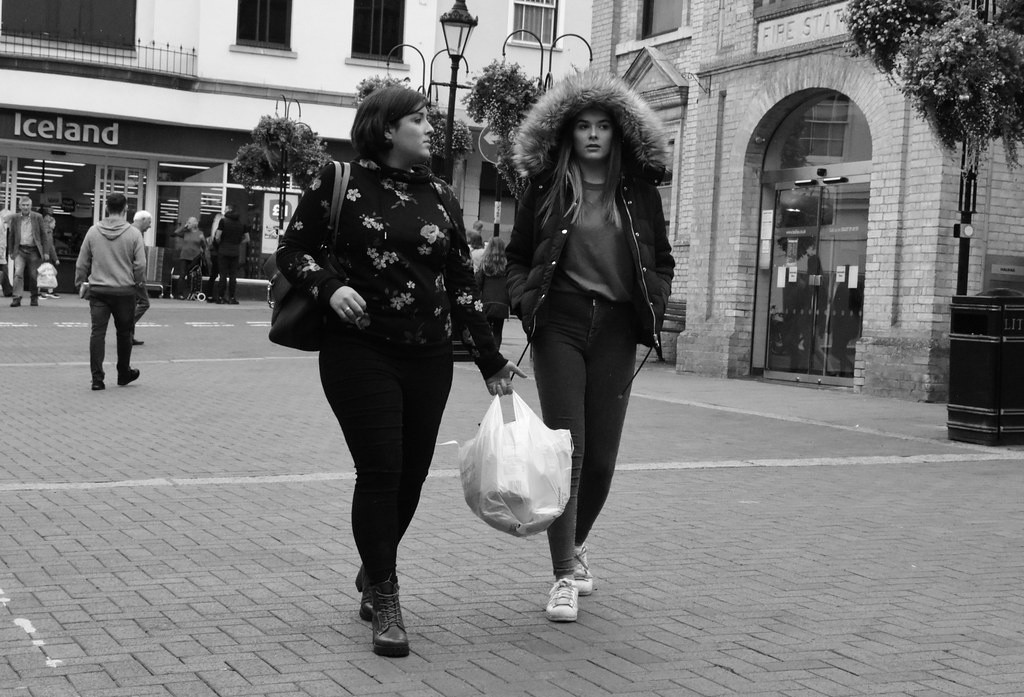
[
  {"x1": 356, "y1": 563, "x2": 375, "y2": 620},
  {"x1": 370, "y1": 581, "x2": 408, "y2": 656}
]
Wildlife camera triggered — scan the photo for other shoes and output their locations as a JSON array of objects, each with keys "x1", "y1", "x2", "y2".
[
  {"x1": 46, "y1": 292, "x2": 60, "y2": 298},
  {"x1": 117, "y1": 369, "x2": 140, "y2": 385},
  {"x1": 174, "y1": 294, "x2": 240, "y2": 304},
  {"x1": 132, "y1": 339, "x2": 144, "y2": 344},
  {"x1": 31, "y1": 295, "x2": 39, "y2": 306},
  {"x1": 37, "y1": 294, "x2": 46, "y2": 299},
  {"x1": 10, "y1": 298, "x2": 21, "y2": 307},
  {"x1": 91, "y1": 381, "x2": 105, "y2": 389}
]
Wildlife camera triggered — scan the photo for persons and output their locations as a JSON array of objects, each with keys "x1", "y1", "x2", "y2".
[
  {"x1": 207, "y1": 209, "x2": 251, "y2": 305},
  {"x1": 0, "y1": 209, "x2": 13, "y2": 297},
  {"x1": 129, "y1": 210, "x2": 150, "y2": 345},
  {"x1": 831, "y1": 264, "x2": 860, "y2": 373},
  {"x1": 506, "y1": 75, "x2": 676, "y2": 622},
  {"x1": 8, "y1": 197, "x2": 49, "y2": 307},
  {"x1": 466, "y1": 220, "x2": 508, "y2": 353},
  {"x1": 802, "y1": 254, "x2": 834, "y2": 375},
  {"x1": 74, "y1": 193, "x2": 146, "y2": 391},
  {"x1": 277, "y1": 88, "x2": 527, "y2": 656},
  {"x1": 37, "y1": 216, "x2": 61, "y2": 300},
  {"x1": 174, "y1": 217, "x2": 206, "y2": 299}
]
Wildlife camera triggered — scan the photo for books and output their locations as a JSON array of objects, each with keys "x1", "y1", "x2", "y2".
[{"x1": 80, "y1": 283, "x2": 91, "y2": 300}]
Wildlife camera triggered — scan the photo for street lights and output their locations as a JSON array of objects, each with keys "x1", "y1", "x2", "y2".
[{"x1": 437, "y1": 0, "x2": 480, "y2": 187}]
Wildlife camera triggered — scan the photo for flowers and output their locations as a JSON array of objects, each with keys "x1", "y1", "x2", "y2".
[
  {"x1": 231, "y1": 115, "x2": 334, "y2": 191},
  {"x1": 355, "y1": 58, "x2": 548, "y2": 200}
]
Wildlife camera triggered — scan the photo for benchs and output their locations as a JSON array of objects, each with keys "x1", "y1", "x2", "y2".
[{"x1": 655, "y1": 298, "x2": 687, "y2": 363}]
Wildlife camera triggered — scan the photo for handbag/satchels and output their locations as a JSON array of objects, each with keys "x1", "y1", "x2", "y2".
[
  {"x1": 461, "y1": 389, "x2": 574, "y2": 538},
  {"x1": 268, "y1": 161, "x2": 350, "y2": 353},
  {"x1": 37, "y1": 261, "x2": 58, "y2": 288}
]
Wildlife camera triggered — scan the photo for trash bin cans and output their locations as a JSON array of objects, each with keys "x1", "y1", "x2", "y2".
[{"x1": 946, "y1": 295, "x2": 1024, "y2": 447}]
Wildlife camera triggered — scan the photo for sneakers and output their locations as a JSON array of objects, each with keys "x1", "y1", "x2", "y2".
[
  {"x1": 545, "y1": 578, "x2": 579, "y2": 620},
  {"x1": 574, "y1": 542, "x2": 594, "y2": 596}
]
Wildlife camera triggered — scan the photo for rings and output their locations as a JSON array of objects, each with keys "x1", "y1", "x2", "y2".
[{"x1": 345, "y1": 308, "x2": 351, "y2": 312}]
